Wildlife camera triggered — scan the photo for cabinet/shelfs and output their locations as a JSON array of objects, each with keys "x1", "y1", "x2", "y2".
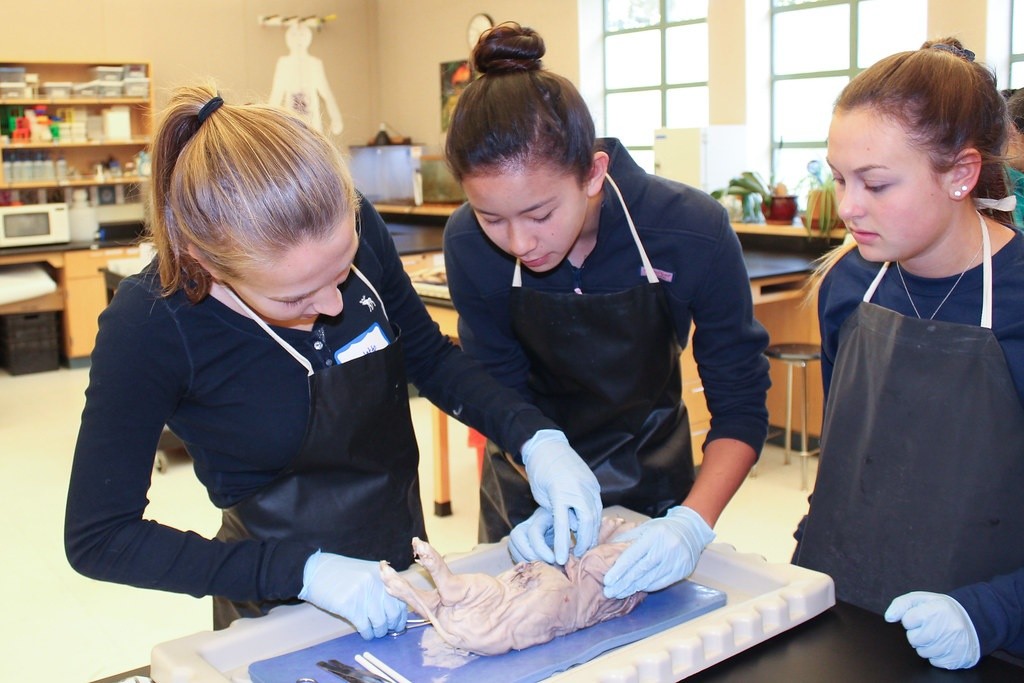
[{"x1": 0, "y1": 62, "x2": 159, "y2": 372}]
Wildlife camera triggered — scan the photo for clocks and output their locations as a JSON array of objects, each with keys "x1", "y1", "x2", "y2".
[{"x1": 465, "y1": 13, "x2": 494, "y2": 51}]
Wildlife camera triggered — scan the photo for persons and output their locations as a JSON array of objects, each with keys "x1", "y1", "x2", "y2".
[
  {"x1": 791, "y1": 37, "x2": 1024, "y2": 667},
  {"x1": 443, "y1": 13, "x2": 771, "y2": 600},
  {"x1": 64, "y1": 85, "x2": 603, "y2": 640}
]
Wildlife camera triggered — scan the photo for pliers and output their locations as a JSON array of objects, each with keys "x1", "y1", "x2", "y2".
[{"x1": 315, "y1": 659, "x2": 390, "y2": 683}]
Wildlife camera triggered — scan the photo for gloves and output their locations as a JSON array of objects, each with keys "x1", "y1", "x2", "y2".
[
  {"x1": 603, "y1": 505, "x2": 717, "y2": 601},
  {"x1": 508, "y1": 507, "x2": 587, "y2": 567},
  {"x1": 297, "y1": 549, "x2": 409, "y2": 641},
  {"x1": 521, "y1": 428, "x2": 610, "y2": 564},
  {"x1": 883, "y1": 592, "x2": 983, "y2": 669}
]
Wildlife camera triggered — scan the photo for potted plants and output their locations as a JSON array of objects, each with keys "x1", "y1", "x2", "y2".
[
  {"x1": 710, "y1": 171, "x2": 797, "y2": 226},
  {"x1": 794, "y1": 175, "x2": 840, "y2": 246}
]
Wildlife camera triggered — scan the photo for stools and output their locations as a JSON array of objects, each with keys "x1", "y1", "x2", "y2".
[{"x1": 749, "y1": 342, "x2": 827, "y2": 494}]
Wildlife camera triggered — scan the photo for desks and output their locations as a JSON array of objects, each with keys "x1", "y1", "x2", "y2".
[
  {"x1": 407, "y1": 244, "x2": 827, "y2": 519},
  {"x1": 89, "y1": 594, "x2": 1024, "y2": 683},
  {"x1": 99, "y1": 223, "x2": 445, "y2": 473}
]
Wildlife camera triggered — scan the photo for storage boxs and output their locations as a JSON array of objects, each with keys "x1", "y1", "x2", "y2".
[
  {"x1": 3, "y1": 312, "x2": 60, "y2": 376},
  {"x1": 39, "y1": 79, "x2": 75, "y2": 100},
  {"x1": 0, "y1": 68, "x2": 39, "y2": 98},
  {"x1": 75, "y1": 69, "x2": 123, "y2": 96},
  {"x1": 120, "y1": 66, "x2": 150, "y2": 98}
]
